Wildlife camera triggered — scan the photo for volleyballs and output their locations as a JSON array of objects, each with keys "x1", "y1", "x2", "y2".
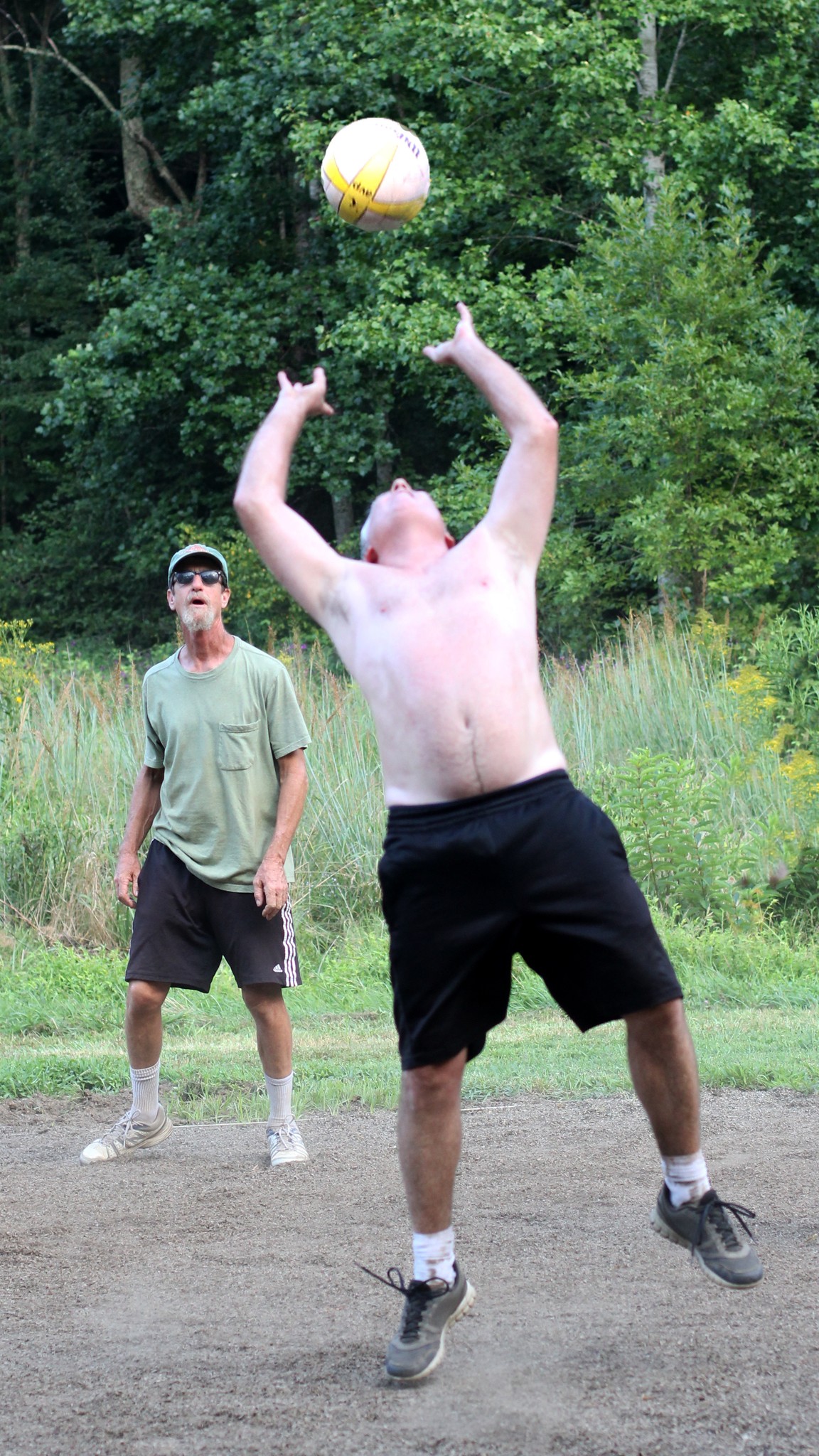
[{"x1": 321, "y1": 117, "x2": 431, "y2": 231}]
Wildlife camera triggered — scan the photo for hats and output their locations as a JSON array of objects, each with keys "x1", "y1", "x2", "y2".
[{"x1": 168, "y1": 543, "x2": 228, "y2": 589}]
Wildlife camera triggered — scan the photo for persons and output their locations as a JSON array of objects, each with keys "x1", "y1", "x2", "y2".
[
  {"x1": 79, "y1": 544, "x2": 315, "y2": 1168},
  {"x1": 233, "y1": 305, "x2": 763, "y2": 1380}
]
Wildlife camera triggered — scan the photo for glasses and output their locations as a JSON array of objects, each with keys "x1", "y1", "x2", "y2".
[{"x1": 170, "y1": 570, "x2": 226, "y2": 590}]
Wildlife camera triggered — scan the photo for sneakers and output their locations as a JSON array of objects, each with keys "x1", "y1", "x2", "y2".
[
  {"x1": 649, "y1": 1179, "x2": 764, "y2": 1290},
  {"x1": 266, "y1": 1114, "x2": 309, "y2": 1167},
  {"x1": 80, "y1": 1103, "x2": 173, "y2": 1166},
  {"x1": 353, "y1": 1259, "x2": 476, "y2": 1382}
]
